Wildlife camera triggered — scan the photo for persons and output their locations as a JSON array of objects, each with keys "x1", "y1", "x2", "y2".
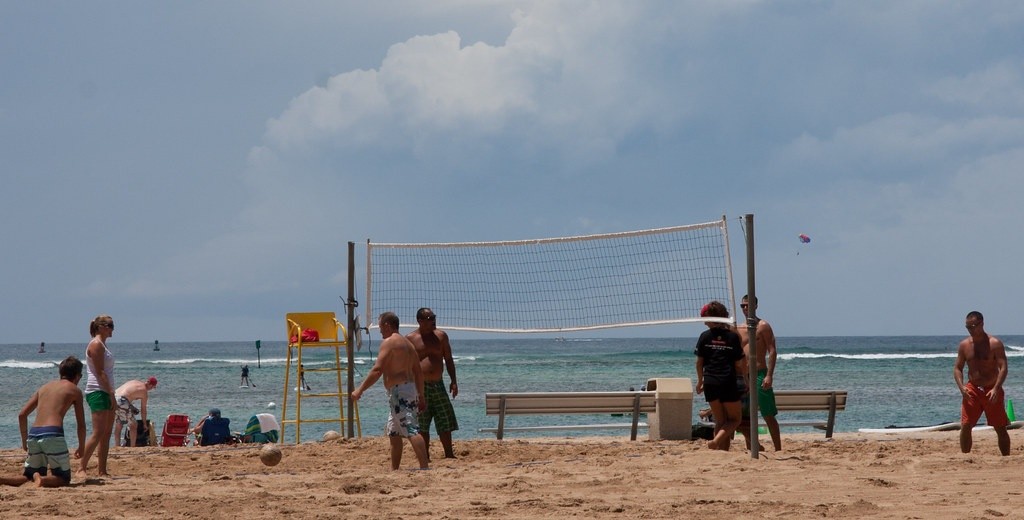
[
  {"x1": 0, "y1": 357, "x2": 86, "y2": 487},
  {"x1": 736, "y1": 295, "x2": 782, "y2": 451},
  {"x1": 114, "y1": 377, "x2": 158, "y2": 447},
  {"x1": 691, "y1": 302, "x2": 745, "y2": 451},
  {"x1": 193, "y1": 407, "x2": 221, "y2": 446},
  {"x1": 300, "y1": 364, "x2": 305, "y2": 389},
  {"x1": 955, "y1": 311, "x2": 1011, "y2": 456},
  {"x1": 351, "y1": 312, "x2": 428, "y2": 470},
  {"x1": 241, "y1": 365, "x2": 249, "y2": 386},
  {"x1": 405, "y1": 308, "x2": 459, "y2": 460},
  {"x1": 75, "y1": 316, "x2": 115, "y2": 477}
]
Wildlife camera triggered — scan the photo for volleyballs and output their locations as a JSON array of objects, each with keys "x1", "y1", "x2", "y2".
[
  {"x1": 259, "y1": 445, "x2": 282, "y2": 466},
  {"x1": 323, "y1": 430, "x2": 340, "y2": 441}
]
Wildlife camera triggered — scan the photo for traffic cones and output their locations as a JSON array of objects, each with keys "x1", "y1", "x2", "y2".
[{"x1": 1006, "y1": 400, "x2": 1017, "y2": 423}]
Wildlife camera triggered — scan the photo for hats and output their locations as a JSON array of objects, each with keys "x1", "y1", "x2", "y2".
[{"x1": 147, "y1": 377, "x2": 157, "y2": 387}]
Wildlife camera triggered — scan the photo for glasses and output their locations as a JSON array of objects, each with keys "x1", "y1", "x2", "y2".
[
  {"x1": 740, "y1": 304, "x2": 757, "y2": 308},
  {"x1": 420, "y1": 315, "x2": 436, "y2": 320},
  {"x1": 965, "y1": 321, "x2": 982, "y2": 329},
  {"x1": 97, "y1": 323, "x2": 114, "y2": 330}
]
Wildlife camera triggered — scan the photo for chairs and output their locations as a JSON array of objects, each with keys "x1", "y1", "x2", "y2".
[
  {"x1": 193, "y1": 418, "x2": 232, "y2": 446},
  {"x1": 155, "y1": 414, "x2": 189, "y2": 446},
  {"x1": 124, "y1": 420, "x2": 157, "y2": 447},
  {"x1": 283, "y1": 310, "x2": 362, "y2": 444},
  {"x1": 234, "y1": 414, "x2": 279, "y2": 444}
]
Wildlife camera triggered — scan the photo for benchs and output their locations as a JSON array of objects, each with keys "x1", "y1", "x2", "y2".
[
  {"x1": 478, "y1": 390, "x2": 655, "y2": 441},
  {"x1": 701, "y1": 390, "x2": 847, "y2": 438}
]
